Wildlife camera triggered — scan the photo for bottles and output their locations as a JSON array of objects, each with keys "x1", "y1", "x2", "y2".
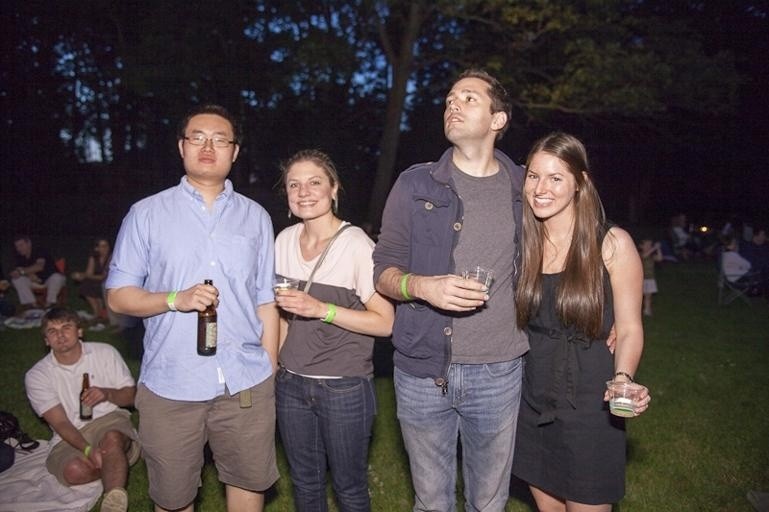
[
  {"x1": 196, "y1": 280, "x2": 217, "y2": 356},
  {"x1": 79, "y1": 374, "x2": 93, "y2": 422}
]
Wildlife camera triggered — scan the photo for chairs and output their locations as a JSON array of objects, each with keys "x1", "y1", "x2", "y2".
[{"x1": 717, "y1": 271, "x2": 754, "y2": 308}]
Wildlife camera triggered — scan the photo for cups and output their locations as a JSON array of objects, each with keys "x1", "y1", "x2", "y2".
[
  {"x1": 271, "y1": 278, "x2": 301, "y2": 296},
  {"x1": 461, "y1": 266, "x2": 495, "y2": 298},
  {"x1": 607, "y1": 381, "x2": 644, "y2": 418}
]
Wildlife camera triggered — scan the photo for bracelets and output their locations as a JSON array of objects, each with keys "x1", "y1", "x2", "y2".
[
  {"x1": 401, "y1": 270, "x2": 413, "y2": 300},
  {"x1": 321, "y1": 302, "x2": 335, "y2": 324},
  {"x1": 83, "y1": 445, "x2": 91, "y2": 458},
  {"x1": 166, "y1": 291, "x2": 177, "y2": 312},
  {"x1": 611, "y1": 372, "x2": 633, "y2": 383}
]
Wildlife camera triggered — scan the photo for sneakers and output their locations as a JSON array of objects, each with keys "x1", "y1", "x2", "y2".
[{"x1": 101, "y1": 486, "x2": 129, "y2": 512}]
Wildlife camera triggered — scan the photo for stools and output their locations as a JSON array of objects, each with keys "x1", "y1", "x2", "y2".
[{"x1": 32, "y1": 285, "x2": 68, "y2": 306}]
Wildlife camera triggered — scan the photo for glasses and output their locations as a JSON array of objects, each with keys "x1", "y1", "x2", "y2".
[{"x1": 184, "y1": 135, "x2": 238, "y2": 150}]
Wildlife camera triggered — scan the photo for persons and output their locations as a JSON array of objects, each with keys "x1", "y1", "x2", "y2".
[
  {"x1": 637, "y1": 211, "x2": 769, "y2": 316},
  {"x1": 371, "y1": 70, "x2": 617, "y2": 512},
  {"x1": 510, "y1": 132, "x2": 652, "y2": 512},
  {"x1": 274, "y1": 150, "x2": 396, "y2": 512},
  {"x1": 103, "y1": 102, "x2": 282, "y2": 512},
  {"x1": 24, "y1": 307, "x2": 140, "y2": 512},
  {"x1": 0, "y1": 233, "x2": 112, "y2": 326}
]
